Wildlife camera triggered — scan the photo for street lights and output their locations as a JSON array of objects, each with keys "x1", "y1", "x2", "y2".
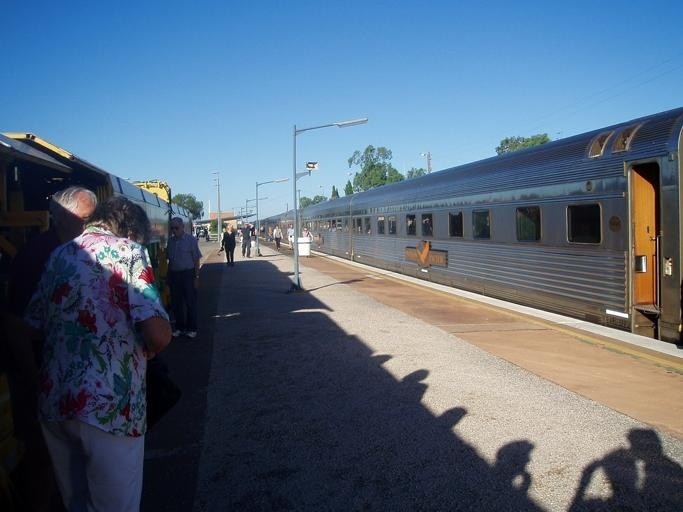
[
  {"x1": 210, "y1": 171, "x2": 220, "y2": 244},
  {"x1": 254, "y1": 177, "x2": 289, "y2": 257},
  {"x1": 291, "y1": 116, "x2": 368, "y2": 294},
  {"x1": 419, "y1": 151, "x2": 432, "y2": 173},
  {"x1": 295, "y1": 189, "x2": 303, "y2": 237},
  {"x1": 245, "y1": 197, "x2": 266, "y2": 223}
]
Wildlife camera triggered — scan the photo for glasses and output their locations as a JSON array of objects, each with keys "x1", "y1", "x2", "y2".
[{"x1": 171, "y1": 226, "x2": 177, "y2": 230}]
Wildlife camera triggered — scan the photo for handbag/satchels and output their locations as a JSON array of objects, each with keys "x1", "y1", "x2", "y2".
[{"x1": 158, "y1": 255, "x2": 168, "y2": 280}]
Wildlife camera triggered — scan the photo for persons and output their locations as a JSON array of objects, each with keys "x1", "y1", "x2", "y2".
[
  {"x1": 195, "y1": 221, "x2": 314, "y2": 266},
  {"x1": 19, "y1": 195, "x2": 173, "y2": 512},
  {"x1": 1, "y1": 184, "x2": 99, "y2": 512},
  {"x1": 158, "y1": 216, "x2": 203, "y2": 338}
]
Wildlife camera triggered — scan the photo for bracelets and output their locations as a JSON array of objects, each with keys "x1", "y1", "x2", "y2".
[{"x1": 193, "y1": 275, "x2": 200, "y2": 279}]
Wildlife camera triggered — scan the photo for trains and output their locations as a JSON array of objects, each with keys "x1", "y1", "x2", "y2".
[
  {"x1": 251, "y1": 108, "x2": 683, "y2": 348},
  {"x1": 0, "y1": 132, "x2": 199, "y2": 355}
]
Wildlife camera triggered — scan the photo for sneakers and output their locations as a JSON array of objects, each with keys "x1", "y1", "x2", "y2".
[
  {"x1": 172, "y1": 328, "x2": 186, "y2": 338},
  {"x1": 185, "y1": 331, "x2": 198, "y2": 338}
]
